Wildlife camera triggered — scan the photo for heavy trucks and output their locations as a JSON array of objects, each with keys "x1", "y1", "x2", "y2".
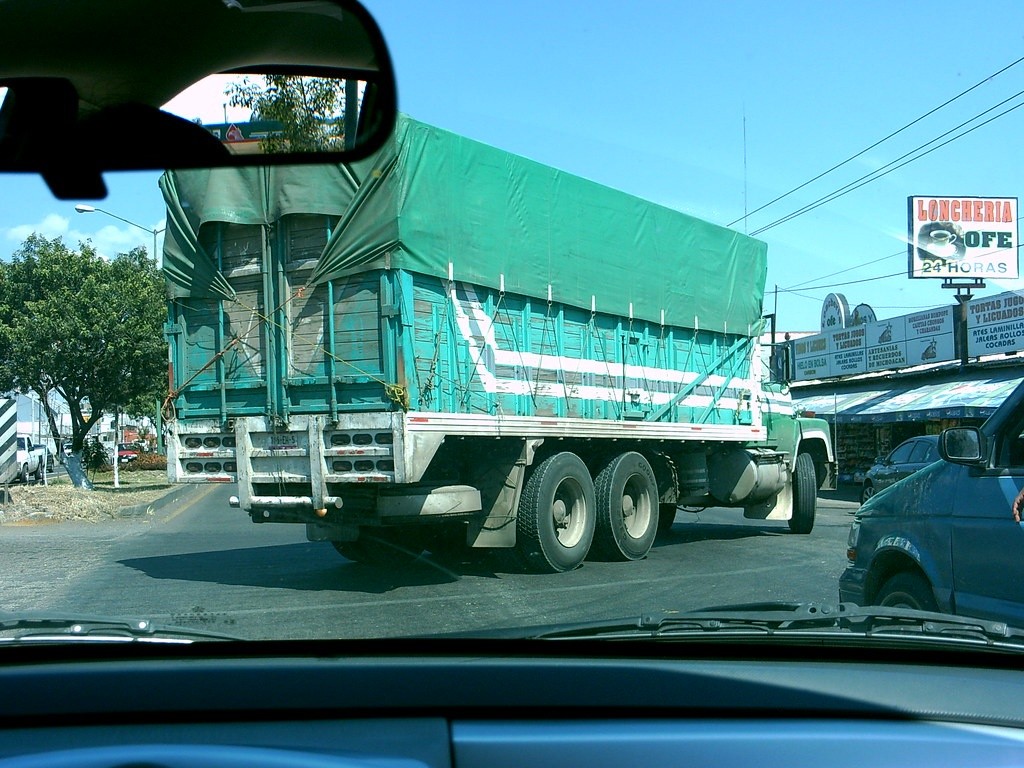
[{"x1": 155, "y1": 106, "x2": 840, "y2": 574}]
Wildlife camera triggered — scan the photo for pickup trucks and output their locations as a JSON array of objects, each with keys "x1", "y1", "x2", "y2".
[{"x1": 10, "y1": 434, "x2": 47, "y2": 486}]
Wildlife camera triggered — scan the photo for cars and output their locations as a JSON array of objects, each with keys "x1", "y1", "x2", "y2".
[
  {"x1": 112, "y1": 442, "x2": 140, "y2": 467},
  {"x1": 859, "y1": 433, "x2": 944, "y2": 510},
  {"x1": 835, "y1": 371, "x2": 1024, "y2": 645},
  {"x1": 32, "y1": 443, "x2": 55, "y2": 472},
  {"x1": 59, "y1": 443, "x2": 74, "y2": 468}
]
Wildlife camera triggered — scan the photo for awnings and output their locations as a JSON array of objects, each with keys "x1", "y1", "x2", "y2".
[
  {"x1": 794, "y1": 389, "x2": 902, "y2": 425},
  {"x1": 854, "y1": 372, "x2": 1024, "y2": 422}
]
